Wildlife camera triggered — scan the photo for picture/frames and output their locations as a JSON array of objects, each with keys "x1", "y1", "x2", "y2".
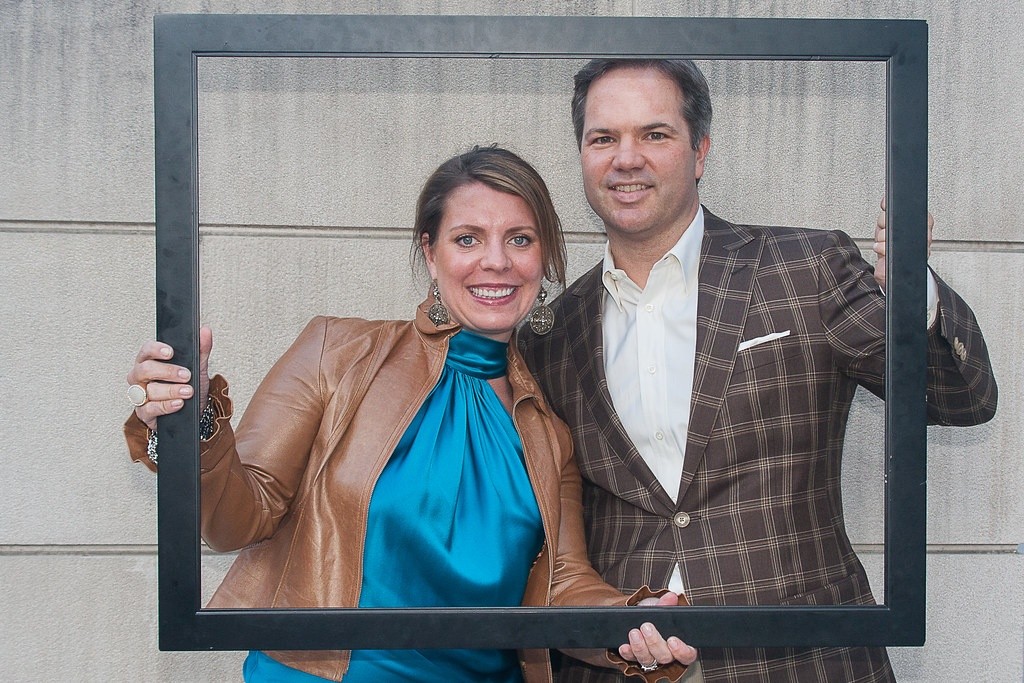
[{"x1": 152, "y1": 13, "x2": 929, "y2": 649}]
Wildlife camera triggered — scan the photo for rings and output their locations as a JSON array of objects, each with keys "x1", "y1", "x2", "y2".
[
  {"x1": 127, "y1": 383, "x2": 149, "y2": 408},
  {"x1": 641, "y1": 659, "x2": 658, "y2": 671}
]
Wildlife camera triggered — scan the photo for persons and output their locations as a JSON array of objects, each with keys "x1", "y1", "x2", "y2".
[
  {"x1": 120, "y1": 144, "x2": 703, "y2": 682},
  {"x1": 518, "y1": 57, "x2": 998, "y2": 682}
]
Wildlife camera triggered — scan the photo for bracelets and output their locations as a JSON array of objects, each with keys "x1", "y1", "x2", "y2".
[{"x1": 147, "y1": 399, "x2": 216, "y2": 466}]
